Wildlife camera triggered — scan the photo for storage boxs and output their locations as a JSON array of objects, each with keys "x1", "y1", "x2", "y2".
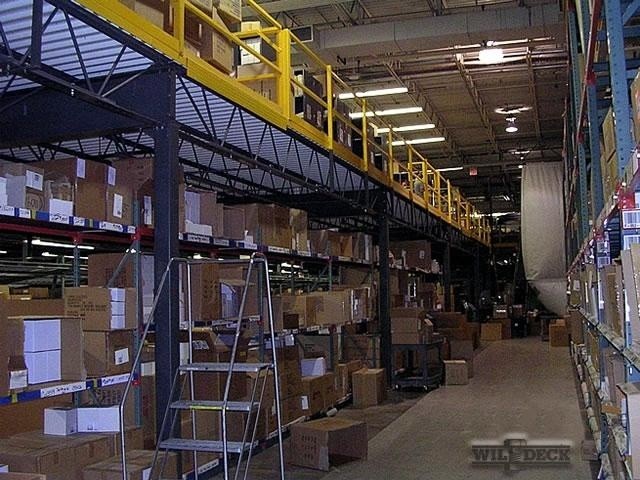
[{"x1": 0, "y1": 1, "x2": 639, "y2": 480}]
